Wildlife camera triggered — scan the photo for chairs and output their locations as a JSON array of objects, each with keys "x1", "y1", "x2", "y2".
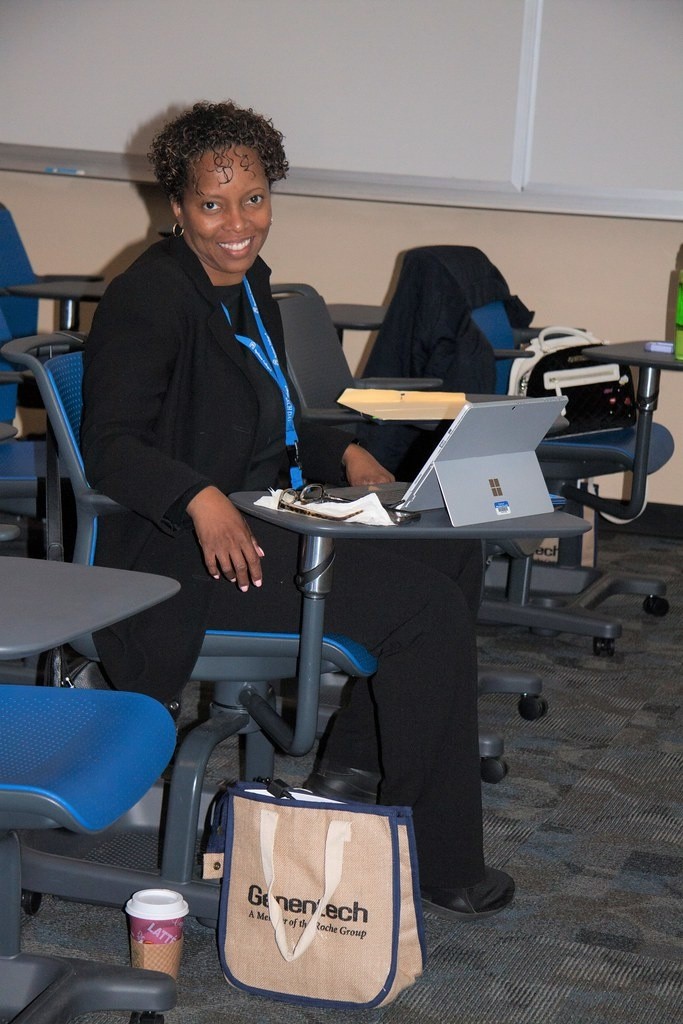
[{"x1": 0, "y1": 202, "x2": 683, "y2": 1023}]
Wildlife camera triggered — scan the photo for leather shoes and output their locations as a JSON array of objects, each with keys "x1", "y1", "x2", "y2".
[
  {"x1": 418, "y1": 859, "x2": 518, "y2": 921},
  {"x1": 304, "y1": 760, "x2": 385, "y2": 806}
]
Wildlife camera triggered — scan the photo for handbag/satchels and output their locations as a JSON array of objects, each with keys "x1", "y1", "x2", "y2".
[
  {"x1": 193, "y1": 777, "x2": 427, "y2": 1011},
  {"x1": 535, "y1": 477, "x2": 603, "y2": 571},
  {"x1": 505, "y1": 327, "x2": 637, "y2": 439}
]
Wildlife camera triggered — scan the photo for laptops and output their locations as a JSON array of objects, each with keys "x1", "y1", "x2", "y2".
[{"x1": 321, "y1": 395, "x2": 570, "y2": 514}]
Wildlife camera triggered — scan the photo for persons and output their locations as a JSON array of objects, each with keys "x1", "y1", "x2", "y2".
[{"x1": 80, "y1": 102, "x2": 519, "y2": 923}]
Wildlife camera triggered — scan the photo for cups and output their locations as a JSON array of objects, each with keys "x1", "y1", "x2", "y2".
[{"x1": 125, "y1": 888, "x2": 191, "y2": 984}]
